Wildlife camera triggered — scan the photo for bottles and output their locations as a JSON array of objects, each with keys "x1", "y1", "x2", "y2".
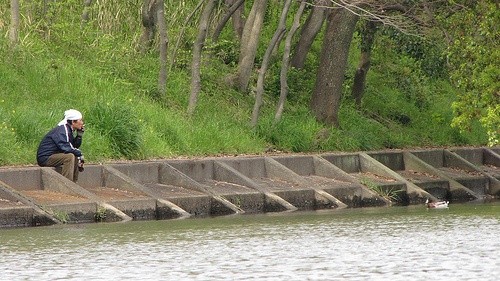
[{"x1": 78, "y1": 157, "x2": 84, "y2": 172}]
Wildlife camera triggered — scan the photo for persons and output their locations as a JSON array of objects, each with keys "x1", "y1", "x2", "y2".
[{"x1": 36, "y1": 108, "x2": 85, "y2": 182}]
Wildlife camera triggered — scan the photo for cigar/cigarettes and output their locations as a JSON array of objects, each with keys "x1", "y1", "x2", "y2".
[{"x1": 82, "y1": 124, "x2": 85, "y2": 126}]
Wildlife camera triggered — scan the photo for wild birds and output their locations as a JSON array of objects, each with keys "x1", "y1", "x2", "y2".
[{"x1": 424, "y1": 198, "x2": 450, "y2": 208}]
